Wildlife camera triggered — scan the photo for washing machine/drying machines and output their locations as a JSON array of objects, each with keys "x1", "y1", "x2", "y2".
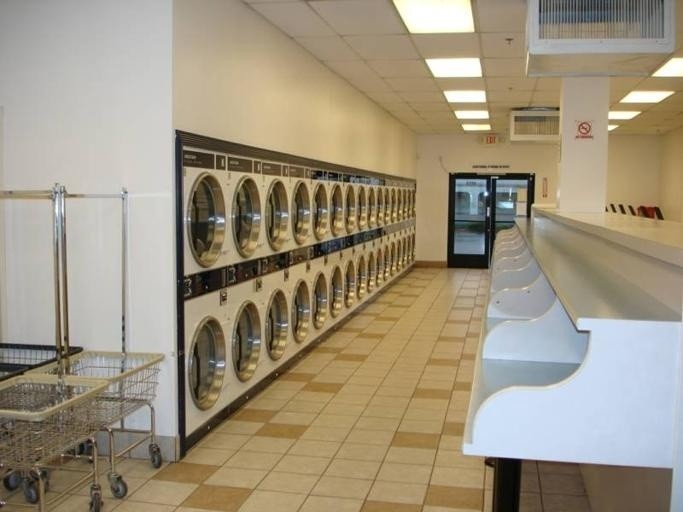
[{"x1": 177, "y1": 129, "x2": 417, "y2": 449}]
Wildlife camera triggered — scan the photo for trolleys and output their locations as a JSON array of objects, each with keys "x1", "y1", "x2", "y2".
[{"x1": 0, "y1": 342, "x2": 163, "y2": 512}]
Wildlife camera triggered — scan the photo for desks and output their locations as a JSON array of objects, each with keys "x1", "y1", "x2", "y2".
[{"x1": 458, "y1": 205, "x2": 683, "y2": 512}]
